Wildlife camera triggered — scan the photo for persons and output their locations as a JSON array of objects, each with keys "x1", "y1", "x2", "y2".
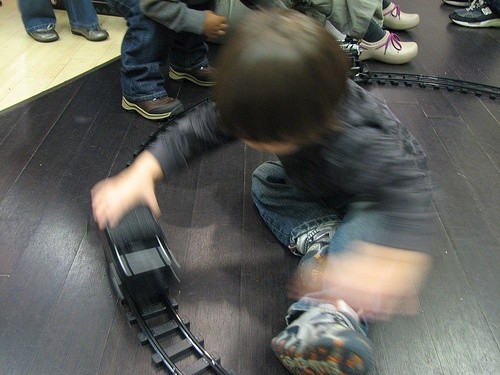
[
  {"x1": 91, "y1": 7, "x2": 438, "y2": 375},
  {"x1": 18, "y1": 0, "x2": 500, "y2": 120}
]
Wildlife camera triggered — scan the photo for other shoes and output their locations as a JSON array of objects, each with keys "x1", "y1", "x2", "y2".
[
  {"x1": 339, "y1": 42, "x2": 370, "y2": 82},
  {"x1": 383, "y1": 2, "x2": 419, "y2": 29},
  {"x1": 357, "y1": 29, "x2": 418, "y2": 64},
  {"x1": 70, "y1": 25, "x2": 109, "y2": 41},
  {"x1": 29, "y1": 29, "x2": 59, "y2": 42}
]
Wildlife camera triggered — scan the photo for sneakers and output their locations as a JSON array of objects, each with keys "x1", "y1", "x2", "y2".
[
  {"x1": 270, "y1": 304, "x2": 375, "y2": 375},
  {"x1": 442, "y1": 0, "x2": 479, "y2": 6},
  {"x1": 448, "y1": 3, "x2": 500, "y2": 27},
  {"x1": 169, "y1": 62, "x2": 218, "y2": 87},
  {"x1": 122, "y1": 94, "x2": 184, "y2": 120},
  {"x1": 298, "y1": 223, "x2": 342, "y2": 265}
]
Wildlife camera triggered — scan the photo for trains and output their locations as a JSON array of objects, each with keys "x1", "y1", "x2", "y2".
[
  {"x1": 103, "y1": 204, "x2": 177, "y2": 299},
  {"x1": 333, "y1": 34, "x2": 370, "y2": 82}
]
